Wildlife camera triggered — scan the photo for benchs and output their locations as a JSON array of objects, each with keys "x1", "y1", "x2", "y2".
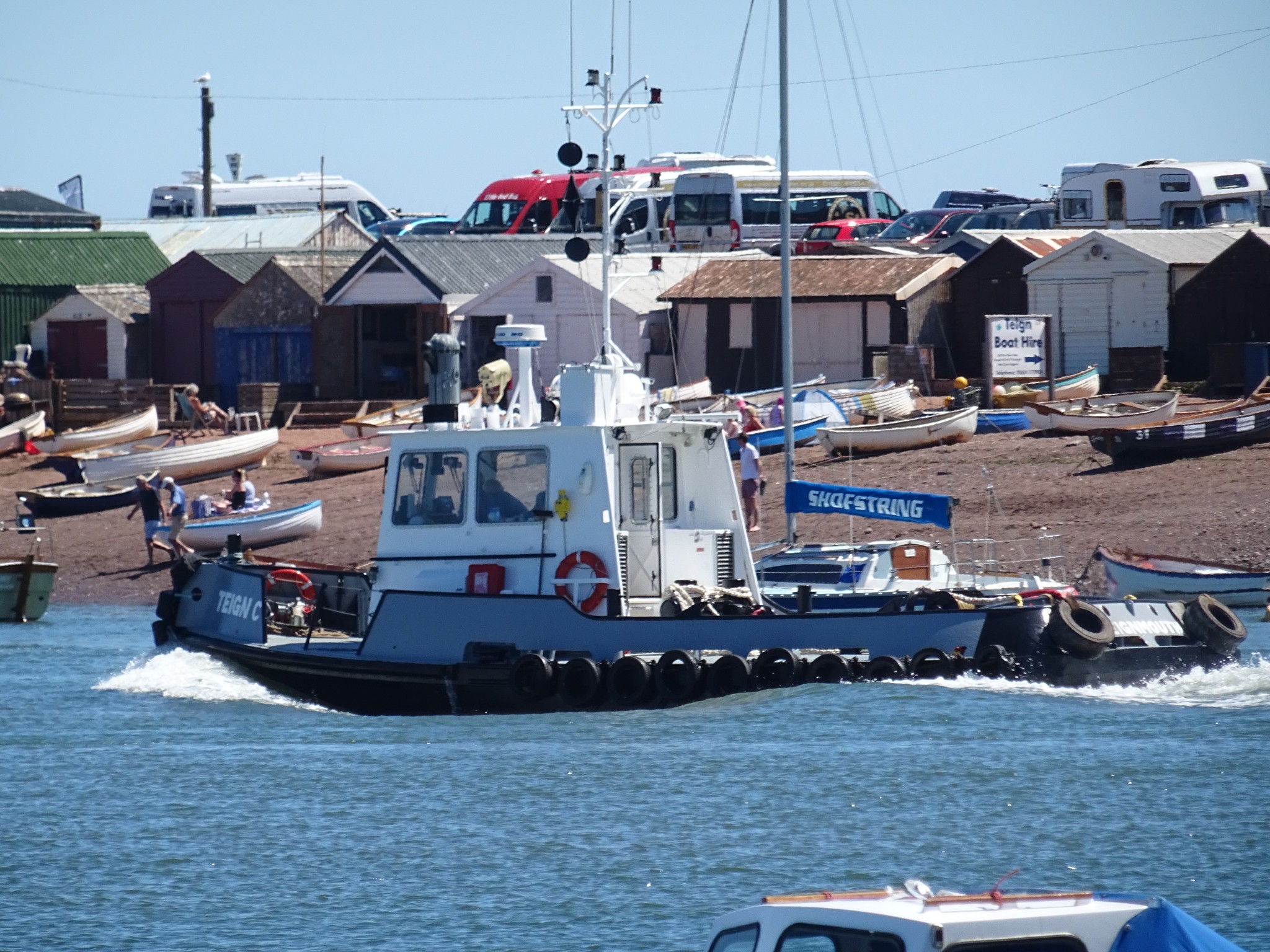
[
  {"x1": 394, "y1": 416, "x2": 423, "y2": 422},
  {"x1": 135, "y1": 443, "x2": 161, "y2": 450},
  {"x1": 330, "y1": 448, "x2": 357, "y2": 456},
  {"x1": 1122, "y1": 402, "x2": 1154, "y2": 411},
  {"x1": 98, "y1": 450, "x2": 116, "y2": 458},
  {"x1": 360, "y1": 446, "x2": 382, "y2": 454},
  {"x1": 1085, "y1": 406, "x2": 1124, "y2": 415},
  {"x1": 106, "y1": 484, "x2": 123, "y2": 491}
]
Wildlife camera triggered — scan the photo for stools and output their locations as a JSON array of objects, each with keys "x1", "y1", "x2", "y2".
[{"x1": 225, "y1": 413, "x2": 262, "y2": 434}]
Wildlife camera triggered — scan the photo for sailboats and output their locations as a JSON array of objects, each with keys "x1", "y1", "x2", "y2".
[{"x1": 151, "y1": 0, "x2": 1269, "y2": 719}]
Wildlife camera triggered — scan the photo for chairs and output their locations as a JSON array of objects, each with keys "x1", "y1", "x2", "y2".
[{"x1": 176, "y1": 392, "x2": 219, "y2": 437}]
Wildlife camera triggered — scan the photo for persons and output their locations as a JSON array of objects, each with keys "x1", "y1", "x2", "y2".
[
  {"x1": 480, "y1": 479, "x2": 531, "y2": 524},
  {"x1": 736, "y1": 400, "x2": 765, "y2": 432},
  {"x1": 185, "y1": 383, "x2": 236, "y2": 434},
  {"x1": 725, "y1": 418, "x2": 743, "y2": 438},
  {"x1": 769, "y1": 397, "x2": 785, "y2": 428},
  {"x1": 161, "y1": 476, "x2": 195, "y2": 561},
  {"x1": 210, "y1": 468, "x2": 255, "y2": 514},
  {"x1": 735, "y1": 432, "x2": 764, "y2": 532},
  {"x1": 126, "y1": 475, "x2": 175, "y2": 568}
]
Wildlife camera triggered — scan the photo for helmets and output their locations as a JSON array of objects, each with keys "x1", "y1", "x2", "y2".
[{"x1": 953, "y1": 376, "x2": 968, "y2": 389}]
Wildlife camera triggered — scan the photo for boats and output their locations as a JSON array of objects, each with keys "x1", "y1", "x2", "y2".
[
  {"x1": 0, "y1": 410, "x2": 47, "y2": 455},
  {"x1": 45, "y1": 433, "x2": 176, "y2": 482},
  {"x1": 814, "y1": 405, "x2": 980, "y2": 459},
  {"x1": 149, "y1": 498, "x2": 325, "y2": 551},
  {"x1": 75, "y1": 426, "x2": 281, "y2": 487},
  {"x1": 1088, "y1": 384, "x2": 1270, "y2": 464},
  {"x1": 973, "y1": 409, "x2": 1030, "y2": 433},
  {"x1": 286, "y1": 433, "x2": 397, "y2": 476},
  {"x1": 0, "y1": 521, "x2": 66, "y2": 626},
  {"x1": 704, "y1": 882, "x2": 1249, "y2": 952},
  {"x1": 633, "y1": 367, "x2": 926, "y2": 460},
  {"x1": 14, "y1": 466, "x2": 163, "y2": 518},
  {"x1": 1016, "y1": 388, "x2": 1185, "y2": 446},
  {"x1": 341, "y1": 385, "x2": 485, "y2": 443},
  {"x1": 1091, "y1": 544, "x2": 1270, "y2": 606},
  {"x1": 31, "y1": 402, "x2": 159, "y2": 455}
]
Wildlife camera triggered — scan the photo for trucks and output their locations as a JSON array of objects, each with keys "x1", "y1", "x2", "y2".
[{"x1": 1055, "y1": 154, "x2": 1270, "y2": 232}]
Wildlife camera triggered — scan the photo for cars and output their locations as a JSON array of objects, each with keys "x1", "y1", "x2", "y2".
[
  {"x1": 793, "y1": 218, "x2": 895, "y2": 247},
  {"x1": 364, "y1": 214, "x2": 458, "y2": 233}
]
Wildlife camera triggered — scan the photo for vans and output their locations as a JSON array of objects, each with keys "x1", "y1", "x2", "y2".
[
  {"x1": 154, "y1": 171, "x2": 400, "y2": 239},
  {"x1": 671, "y1": 170, "x2": 914, "y2": 251},
  {"x1": 452, "y1": 167, "x2": 690, "y2": 236}
]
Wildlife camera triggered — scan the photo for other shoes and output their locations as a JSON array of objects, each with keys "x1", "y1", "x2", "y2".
[{"x1": 746, "y1": 526, "x2": 761, "y2": 532}]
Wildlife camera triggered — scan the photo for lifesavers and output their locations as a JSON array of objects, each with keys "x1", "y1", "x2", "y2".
[
  {"x1": 861, "y1": 656, "x2": 908, "y2": 681},
  {"x1": 607, "y1": 655, "x2": 652, "y2": 706},
  {"x1": 508, "y1": 653, "x2": 556, "y2": 702},
  {"x1": 753, "y1": 647, "x2": 799, "y2": 690},
  {"x1": 555, "y1": 551, "x2": 608, "y2": 613},
  {"x1": 1047, "y1": 597, "x2": 1116, "y2": 654},
  {"x1": 1181, "y1": 594, "x2": 1249, "y2": 655},
  {"x1": 265, "y1": 569, "x2": 316, "y2": 606},
  {"x1": 805, "y1": 653, "x2": 855, "y2": 684},
  {"x1": 972, "y1": 643, "x2": 1009, "y2": 680},
  {"x1": 559, "y1": 657, "x2": 602, "y2": 706},
  {"x1": 711, "y1": 654, "x2": 751, "y2": 696},
  {"x1": 908, "y1": 647, "x2": 953, "y2": 679},
  {"x1": 654, "y1": 649, "x2": 701, "y2": 700}
]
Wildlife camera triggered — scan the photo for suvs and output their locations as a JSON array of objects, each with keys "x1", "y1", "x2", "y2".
[{"x1": 831, "y1": 190, "x2": 1057, "y2": 248}]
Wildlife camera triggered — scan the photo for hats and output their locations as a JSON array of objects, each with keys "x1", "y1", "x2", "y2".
[
  {"x1": 135, "y1": 475, "x2": 147, "y2": 485},
  {"x1": 777, "y1": 397, "x2": 784, "y2": 405},
  {"x1": 161, "y1": 477, "x2": 174, "y2": 488}
]
